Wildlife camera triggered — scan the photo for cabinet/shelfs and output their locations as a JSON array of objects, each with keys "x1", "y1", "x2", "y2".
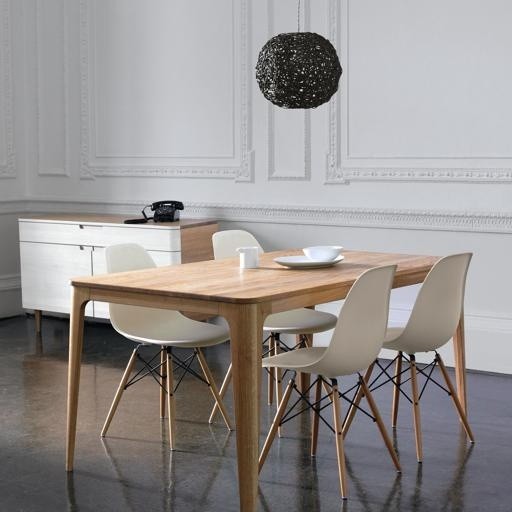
[{"x1": 18, "y1": 219, "x2": 219, "y2": 334}]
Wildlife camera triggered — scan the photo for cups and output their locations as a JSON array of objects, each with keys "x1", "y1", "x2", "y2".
[{"x1": 237, "y1": 247, "x2": 259, "y2": 269}]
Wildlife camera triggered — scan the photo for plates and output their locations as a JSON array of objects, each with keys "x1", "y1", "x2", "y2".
[{"x1": 273, "y1": 255, "x2": 346, "y2": 268}]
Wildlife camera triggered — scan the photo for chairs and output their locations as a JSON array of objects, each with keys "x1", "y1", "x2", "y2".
[
  {"x1": 342, "y1": 252, "x2": 475, "y2": 464},
  {"x1": 99, "y1": 242, "x2": 233, "y2": 451},
  {"x1": 207, "y1": 230, "x2": 337, "y2": 439},
  {"x1": 259, "y1": 264, "x2": 403, "y2": 501}
]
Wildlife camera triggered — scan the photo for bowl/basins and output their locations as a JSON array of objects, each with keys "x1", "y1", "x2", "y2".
[{"x1": 303, "y1": 246, "x2": 344, "y2": 261}]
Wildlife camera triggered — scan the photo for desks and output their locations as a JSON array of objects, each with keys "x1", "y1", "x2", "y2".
[{"x1": 65, "y1": 247, "x2": 467, "y2": 512}]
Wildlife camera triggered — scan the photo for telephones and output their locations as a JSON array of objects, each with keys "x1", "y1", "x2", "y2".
[{"x1": 151, "y1": 200, "x2": 184, "y2": 223}]
